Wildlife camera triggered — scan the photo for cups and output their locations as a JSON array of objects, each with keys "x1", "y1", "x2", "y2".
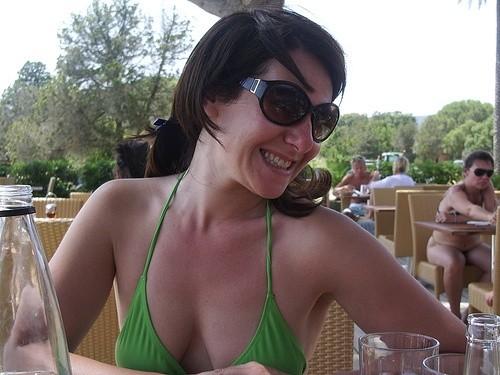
[
  {"x1": 422, "y1": 353, "x2": 497, "y2": 375},
  {"x1": 361, "y1": 184, "x2": 367, "y2": 196},
  {"x1": 358, "y1": 331, "x2": 440, "y2": 375}
]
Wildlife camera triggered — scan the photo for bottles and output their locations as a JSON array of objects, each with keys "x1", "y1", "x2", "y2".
[
  {"x1": 462, "y1": 312, "x2": 500, "y2": 375},
  {"x1": 0, "y1": 185, "x2": 72, "y2": 375},
  {"x1": 45, "y1": 190, "x2": 57, "y2": 218}
]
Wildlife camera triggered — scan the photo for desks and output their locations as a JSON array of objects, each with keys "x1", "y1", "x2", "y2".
[
  {"x1": 332, "y1": 190, "x2": 370, "y2": 205},
  {"x1": 364, "y1": 205, "x2": 395, "y2": 212},
  {"x1": 413, "y1": 220, "x2": 497, "y2": 277}
]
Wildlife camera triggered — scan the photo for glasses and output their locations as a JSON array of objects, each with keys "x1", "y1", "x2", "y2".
[
  {"x1": 237, "y1": 77, "x2": 340, "y2": 143},
  {"x1": 468, "y1": 168, "x2": 494, "y2": 177}
]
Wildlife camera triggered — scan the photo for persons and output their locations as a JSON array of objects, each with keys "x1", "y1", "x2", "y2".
[
  {"x1": 113, "y1": 138, "x2": 151, "y2": 178},
  {"x1": 367, "y1": 156, "x2": 416, "y2": 220},
  {"x1": 3, "y1": 6, "x2": 484, "y2": 375},
  {"x1": 427, "y1": 151, "x2": 498, "y2": 324},
  {"x1": 334, "y1": 155, "x2": 372, "y2": 214}
]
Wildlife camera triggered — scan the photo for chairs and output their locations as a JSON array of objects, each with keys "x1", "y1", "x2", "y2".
[
  {"x1": 0, "y1": 192, "x2": 354, "y2": 375},
  {"x1": 370, "y1": 181, "x2": 500, "y2": 318}
]
